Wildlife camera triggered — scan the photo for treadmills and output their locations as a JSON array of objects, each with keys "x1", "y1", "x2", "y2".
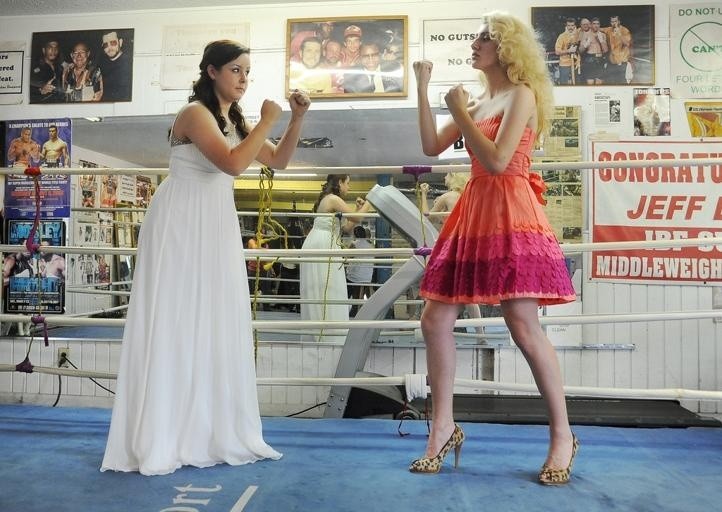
[{"x1": 322, "y1": 183, "x2": 721, "y2": 428}]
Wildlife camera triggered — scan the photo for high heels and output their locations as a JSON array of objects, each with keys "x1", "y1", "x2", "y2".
[
  {"x1": 410, "y1": 423, "x2": 465, "y2": 473},
  {"x1": 539, "y1": 431, "x2": 580, "y2": 486}
]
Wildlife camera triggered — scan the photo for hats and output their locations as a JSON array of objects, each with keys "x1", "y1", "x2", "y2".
[{"x1": 344, "y1": 25, "x2": 362, "y2": 37}]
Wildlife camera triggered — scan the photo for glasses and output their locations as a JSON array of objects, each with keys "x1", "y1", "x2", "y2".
[{"x1": 102, "y1": 40, "x2": 117, "y2": 49}]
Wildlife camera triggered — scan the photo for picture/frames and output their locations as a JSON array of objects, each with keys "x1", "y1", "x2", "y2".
[
  {"x1": 283, "y1": 14, "x2": 410, "y2": 101},
  {"x1": 417, "y1": 15, "x2": 491, "y2": 86},
  {"x1": 526, "y1": 4, "x2": 660, "y2": 87}
]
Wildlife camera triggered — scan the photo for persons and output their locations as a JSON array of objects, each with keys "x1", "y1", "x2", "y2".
[
  {"x1": 576, "y1": 17, "x2": 591, "y2": 36},
  {"x1": 555, "y1": 17, "x2": 578, "y2": 84},
  {"x1": 297, "y1": 173, "x2": 374, "y2": 343},
  {"x1": 422, "y1": 174, "x2": 488, "y2": 345},
  {"x1": 406, "y1": 14, "x2": 580, "y2": 486},
  {"x1": 98, "y1": 38, "x2": 310, "y2": 479},
  {"x1": 599, "y1": 15, "x2": 634, "y2": 82},
  {"x1": 242, "y1": 226, "x2": 375, "y2": 319},
  {"x1": 288, "y1": 19, "x2": 404, "y2": 97},
  {"x1": 99, "y1": 30, "x2": 131, "y2": 100},
  {"x1": 59, "y1": 41, "x2": 103, "y2": 101},
  {"x1": 29, "y1": 238, "x2": 65, "y2": 282},
  {"x1": 30, "y1": 38, "x2": 62, "y2": 99},
  {"x1": 1, "y1": 238, "x2": 34, "y2": 287},
  {"x1": 580, "y1": 17, "x2": 610, "y2": 84},
  {"x1": 38, "y1": 125, "x2": 70, "y2": 168},
  {"x1": 2, "y1": 126, "x2": 41, "y2": 177}
]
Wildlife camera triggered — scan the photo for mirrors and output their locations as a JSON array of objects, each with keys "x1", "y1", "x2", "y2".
[{"x1": 111, "y1": 201, "x2": 136, "y2": 314}]
